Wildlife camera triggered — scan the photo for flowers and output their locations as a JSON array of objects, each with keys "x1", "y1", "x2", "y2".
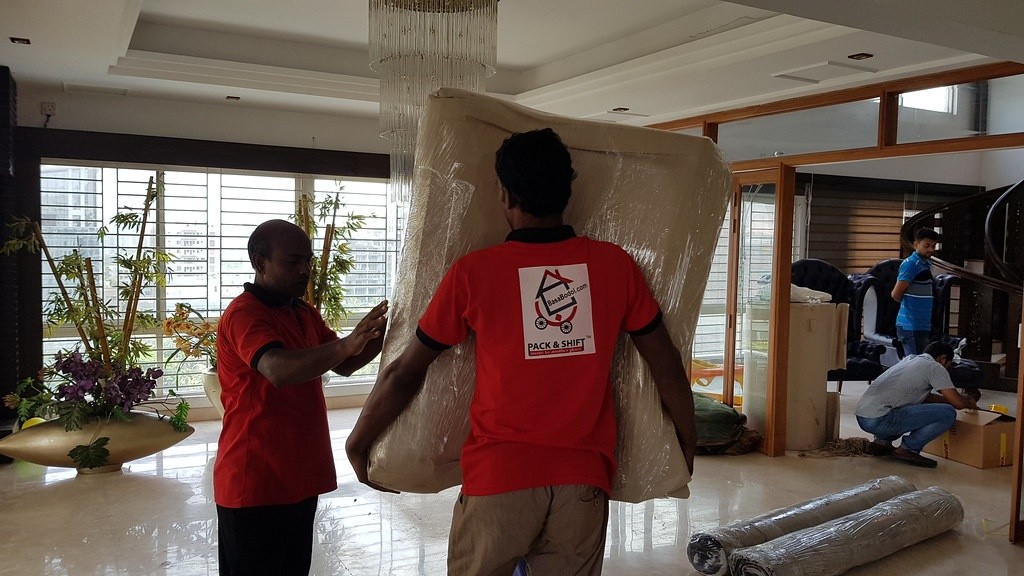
[
  {"x1": 163, "y1": 303, "x2": 225, "y2": 392},
  {"x1": 0, "y1": 169, "x2": 196, "y2": 470}
]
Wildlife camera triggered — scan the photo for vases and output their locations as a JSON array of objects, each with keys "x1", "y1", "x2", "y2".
[
  {"x1": 204, "y1": 368, "x2": 225, "y2": 420},
  {"x1": 0, "y1": 409, "x2": 195, "y2": 475}
]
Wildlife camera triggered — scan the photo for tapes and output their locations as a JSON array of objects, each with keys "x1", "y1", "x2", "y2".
[{"x1": 989, "y1": 404, "x2": 1008, "y2": 414}]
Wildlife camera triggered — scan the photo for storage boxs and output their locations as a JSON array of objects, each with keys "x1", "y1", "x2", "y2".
[{"x1": 920, "y1": 405, "x2": 1016, "y2": 470}]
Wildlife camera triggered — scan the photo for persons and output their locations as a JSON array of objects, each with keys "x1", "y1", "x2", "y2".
[
  {"x1": 891, "y1": 228, "x2": 940, "y2": 356},
  {"x1": 214, "y1": 219, "x2": 388, "y2": 576},
  {"x1": 345, "y1": 128, "x2": 696, "y2": 576},
  {"x1": 854, "y1": 341, "x2": 982, "y2": 467}
]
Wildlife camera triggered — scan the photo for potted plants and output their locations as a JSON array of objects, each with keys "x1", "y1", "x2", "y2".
[{"x1": 286, "y1": 174, "x2": 377, "y2": 393}]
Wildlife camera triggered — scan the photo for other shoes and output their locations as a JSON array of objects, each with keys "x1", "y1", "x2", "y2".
[
  {"x1": 866, "y1": 442, "x2": 898, "y2": 456},
  {"x1": 892, "y1": 446, "x2": 937, "y2": 467}
]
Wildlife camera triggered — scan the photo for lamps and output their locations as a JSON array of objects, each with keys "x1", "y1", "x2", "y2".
[{"x1": 366, "y1": 0, "x2": 499, "y2": 205}]
